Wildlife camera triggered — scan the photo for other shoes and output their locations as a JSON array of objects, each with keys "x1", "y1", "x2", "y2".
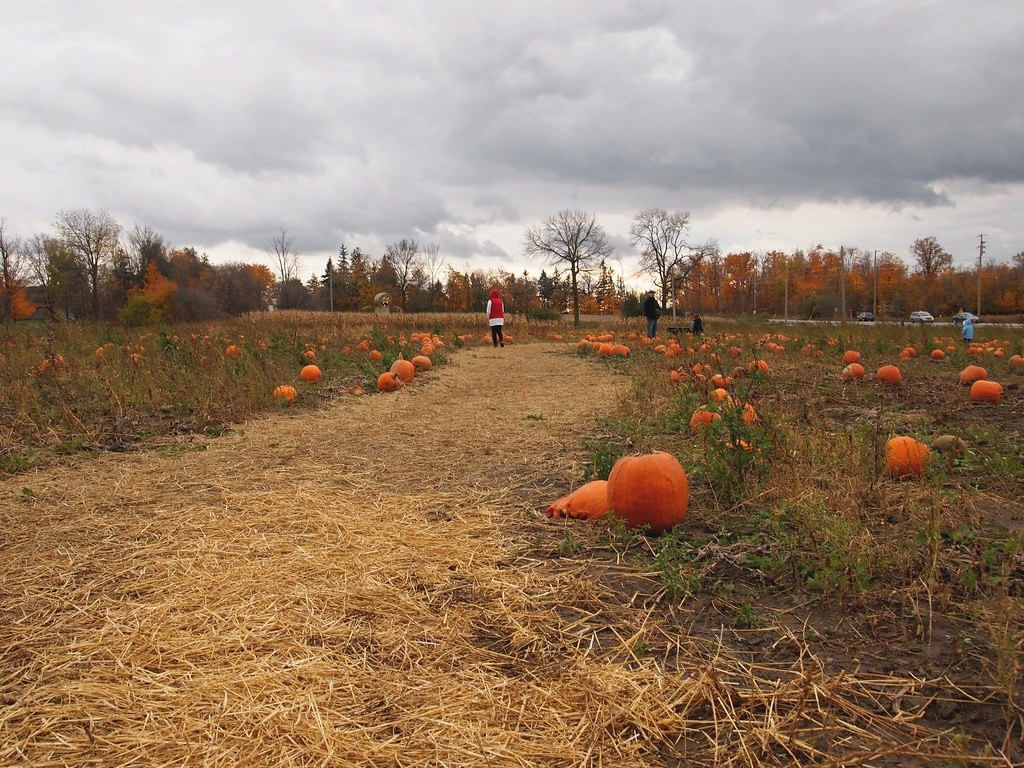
[
  {"x1": 501, "y1": 343, "x2": 504, "y2": 347},
  {"x1": 494, "y1": 343, "x2": 498, "y2": 348}
]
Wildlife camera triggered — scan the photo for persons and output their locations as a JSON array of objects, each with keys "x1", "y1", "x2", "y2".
[
  {"x1": 644, "y1": 290, "x2": 661, "y2": 339},
  {"x1": 690, "y1": 313, "x2": 704, "y2": 335},
  {"x1": 959, "y1": 307, "x2": 964, "y2": 313},
  {"x1": 486, "y1": 289, "x2": 505, "y2": 347},
  {"x1": 961, "y1": 319, "x2": 974, "y2": 342}
]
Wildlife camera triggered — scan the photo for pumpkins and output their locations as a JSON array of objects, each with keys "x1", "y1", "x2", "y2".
[
  {"x1": 5, "y1": 334, "x2": 515, "y2": 399},
  {"x1": 544, "y1": 333, "x2": 1024, "y2": 532}
]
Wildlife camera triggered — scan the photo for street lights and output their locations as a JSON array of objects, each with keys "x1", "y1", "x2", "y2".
[{"x1": 874, "y1": 249, "x2": 882, "y2": 322}]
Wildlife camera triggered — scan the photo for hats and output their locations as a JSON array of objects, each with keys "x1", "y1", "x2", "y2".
[
  {"x1": 648, "y1": 290, "x2": 657, "y2": 294},
  {"x1": 692, "y1": 313, "x2": 698, "y2": 318}
]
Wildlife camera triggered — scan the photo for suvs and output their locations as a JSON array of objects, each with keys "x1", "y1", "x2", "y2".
[
  {"x1": 909, "y1": 311, "x2": 934, "y2": 325},
  {"x1": 952, "y1": 312, "x2": 980, "y2": 324},
  {"x1": 856, "y1": 311, "x2": 874, "y2": 322}
]
